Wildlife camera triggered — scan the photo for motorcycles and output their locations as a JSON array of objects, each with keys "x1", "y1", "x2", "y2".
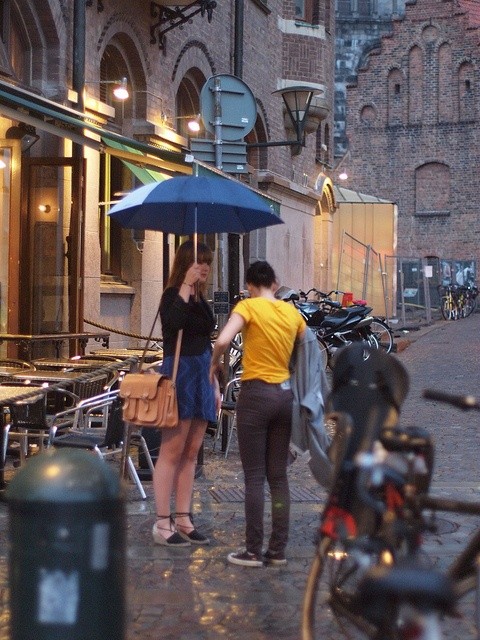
[{"x1": 273, "y1": 286, "x2": 366, "y2": 371}]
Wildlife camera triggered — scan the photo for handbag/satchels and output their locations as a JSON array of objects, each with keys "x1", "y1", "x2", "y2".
[{"x1": 120, "y1": 283, "x2": 183, "y2": 428}]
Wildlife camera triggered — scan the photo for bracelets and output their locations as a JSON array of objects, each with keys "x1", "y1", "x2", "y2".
[{"x1": 182, "y1": 282, "x2": 193, "y2": 288}]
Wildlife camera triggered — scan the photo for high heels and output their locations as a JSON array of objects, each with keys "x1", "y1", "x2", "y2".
[
  {"x1": 174, "y1": 512, "x2": 211, "y2": 545},
  {"x1": 152, "y1": 515, "x2": 191, "y2": 547}
]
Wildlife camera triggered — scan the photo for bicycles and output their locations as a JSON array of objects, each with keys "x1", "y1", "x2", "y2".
[
  {"x1": 436, "y1": 282, "x2": 478, "y2": 320},
  {"x1": 210, "y1": 337, "x2": 242, "y2": 453},
  {"x1": 314, "y1": 290, "x2": 393, "y2": 353},
  {"x1": 299, "y1": 300, "x2": 371, "y2": 361},
  {"x1": 297, "y1": 287, "x2": 378, "y2": 351},
  {"x1": 301, "y1": 386, "x2": 480, "y2": 640}
]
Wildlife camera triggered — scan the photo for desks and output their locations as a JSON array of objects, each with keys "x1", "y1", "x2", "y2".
[
  {"x1": 32, "y1": 356, "x2": 149, "y2": 373},
  {"x1": 0, "y1": 365, "x2": 88, "y2": 381},
  {"x1": 1, "y1": 386, "x2": 44, "y2": 490},
  {"x1": 90, "y1": 348, "x2": 158, "y2": 358}
]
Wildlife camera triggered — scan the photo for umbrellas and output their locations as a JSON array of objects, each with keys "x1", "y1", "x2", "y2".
[{"x1": 106, "y1": 162, "x2": 285, "y2": 312}]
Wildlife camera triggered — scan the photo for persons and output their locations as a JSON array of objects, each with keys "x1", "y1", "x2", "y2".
[
  {"x1": 209, "y1": 260, "x2": 308, "y2": 567},
  {"x1": 152, "y1": 239, "x2": 217, "y2": 547}
]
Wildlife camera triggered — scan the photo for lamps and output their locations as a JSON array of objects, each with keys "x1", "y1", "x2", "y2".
[
  {"x1": 163, "y1": 113, "x2": 202, "y2": 132},
  {"x1": 245, "y1": 84, "x2": 324, "y2": 150},
  {"x1": 39, "y1": 205, "x2": 51, "y2": 213},
  {"x1": 85, "y1": 78, "x2": 129, "y2": 101},
  {"x1": 327, "y1": 165, "x2": 349, "y2": 181}
]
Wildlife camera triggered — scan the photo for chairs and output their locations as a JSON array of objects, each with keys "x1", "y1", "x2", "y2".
[
  {"x1": 0, "y1": 357, "x2": 39, "y2": 456},
  {"x1": 214, "y1": 374, "x2": 243, "y2": 454},
  {"x1": 0, "y1": 381, "x2": 75, "y2": 468},
  {"x1": 61, "y1": 365, "x2": 118, "y2": 428},
  {"x1": 47, "y1": 399, "x2": 146, "y2": 502},
  {"x1": 72, "y1": 387, "x2": 155, "y2": 472}
]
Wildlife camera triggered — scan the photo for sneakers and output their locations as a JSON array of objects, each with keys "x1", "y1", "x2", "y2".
[
  {"x1": 227, "y1": 549, "x2": 263, "y2": 568},
  {"x1": 260, "y1": 550, "x2": 288, "y2": 565}
]
